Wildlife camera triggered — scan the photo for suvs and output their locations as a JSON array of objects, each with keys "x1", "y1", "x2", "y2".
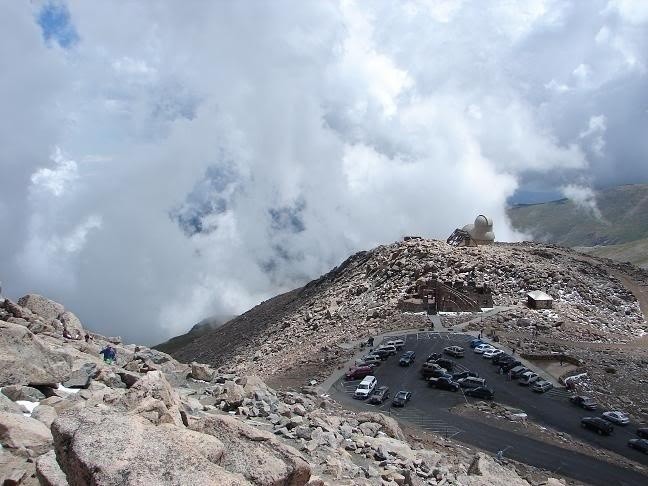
[
  {"x1": 443, "y1": 345, "x2": 465, "y2": 358},
  {"x1": 581, "y1": 416, "x2": 614, "y2": 435},
  {"x1": 370, "y1": 385, "x2": 391, "y2": 404},
  {"x1": 399, "y1": 350, "x2": 416, "y2": 367}
]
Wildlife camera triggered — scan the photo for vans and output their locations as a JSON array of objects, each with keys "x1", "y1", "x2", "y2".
[{"x1": 354, "y1": 375, "x2": 377, "y2": 399}]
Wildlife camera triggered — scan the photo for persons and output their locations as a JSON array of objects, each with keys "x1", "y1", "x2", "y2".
[
  {"x1": 369, "y1": 335, "x2": 374, "y2": 346},
  {"x1": 560, "y1": 357, "x2": 563, "y2": 366},
  {"x1": 478, "y1": 334, "x2": 481, "y2": 340},
  {"x1": 506, "y1": 368, "x2": 511, "y2": 381}
]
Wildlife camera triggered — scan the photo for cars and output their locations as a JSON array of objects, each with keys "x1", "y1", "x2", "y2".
[
  {"x1": 574, "y1": 395, "x2": 598, "y2": 410},
  {"x1": 602, "y1": 411, "x2": 629, "y2": 425},
  {"x1": 627, "y1": 427, "x2": 648, "y2": 455},
  {"x1": 391, "y1": 390, "x2": 412, "y2": 408},
  {"x1": 470, "y1": 338, "x2": 553, "y2": 393},
  {"x1": 421, "y1": 351, "x2": 495, "y2": 400},
  {"x1": 345, "y1": 338, "x2": 405, "y2": 381}
]
